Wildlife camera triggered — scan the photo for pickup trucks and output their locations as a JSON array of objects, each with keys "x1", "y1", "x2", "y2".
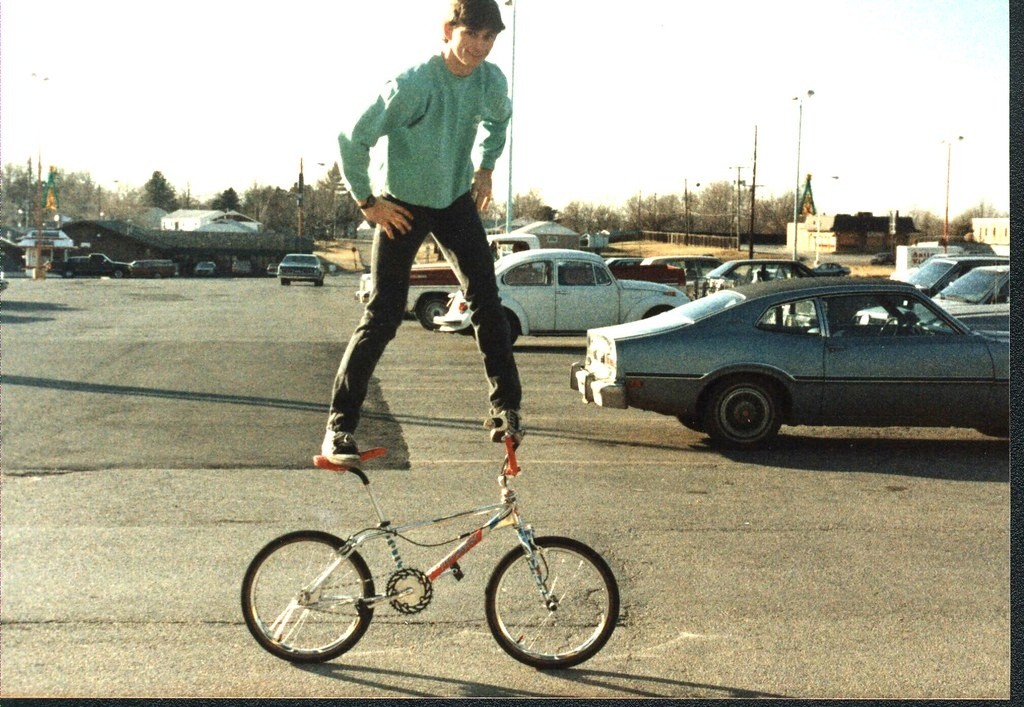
[
  {"x1": 355, "y1": 230, "x2": 687, "y2": 333},
  {"x1": 62, "y1": 252, "x2": 132, "y2": 279},
  {"x1": 129, "y1": 258, "x2": 175, "y2": 279}
]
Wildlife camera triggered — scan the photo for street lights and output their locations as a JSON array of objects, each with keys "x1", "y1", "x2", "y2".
[
  {"x1": 941, "y1": 136, "x2": 965, "y2": 254},
  {"x1": 729, "y1": 165, "x2": 748, "y2": 251},
  {"x1": 792, "y1": 90, "x2": 814, "y2": 262}
]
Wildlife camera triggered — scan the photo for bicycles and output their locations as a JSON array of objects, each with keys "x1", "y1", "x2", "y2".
[{"x1": 240, "y1": 428, "x2": 620, "y2": 670}]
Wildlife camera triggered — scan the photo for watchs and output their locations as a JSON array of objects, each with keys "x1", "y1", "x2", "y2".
[{"x1": 358, "y1": 196, "x2": 376, "y2": 209}]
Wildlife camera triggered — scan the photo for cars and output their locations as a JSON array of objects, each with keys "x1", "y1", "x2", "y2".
[
  {"x1": 810, "y1": 262, "x2": 851, "y2": 277},
  {"x1": 45, "y1": 260, "x2": 62, "y2": 274},
  {"x1": 853, "y1": 253, "x2": 1009, "y2": 328},
  {"x1": 603, "y1": 254, "x2": 821, "y2": 303},
  {"x1": 433, "y1": 248, "x2": 691, "y2": 347},
  {"x1": 277, "y1": 253, "x2": 325, "y2": 287},
  {"x1": 569, "y1": 272, "x2": 1010, "y2": 452},
  {"x1": 193, "y1": 261, "x2": 218, "y2": 276},
  {"x1": 908, "y1": 263, "x2": 1011, "y2": 347},
  {"x1": 266, "y1": 262, "x2": 280, "y2": 275}
]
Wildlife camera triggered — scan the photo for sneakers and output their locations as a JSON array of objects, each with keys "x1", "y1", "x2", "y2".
[
  {"x1": 322, "y1": 430, "x2": 361, "y2": 467},
  {"x1": 483, "y1": 407, "x2": 523, "y2": 445}
]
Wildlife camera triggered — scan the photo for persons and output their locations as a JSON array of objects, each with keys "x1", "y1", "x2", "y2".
[{"x1": 321, "y1": 1, "x2": 523, "y2": 465}]
